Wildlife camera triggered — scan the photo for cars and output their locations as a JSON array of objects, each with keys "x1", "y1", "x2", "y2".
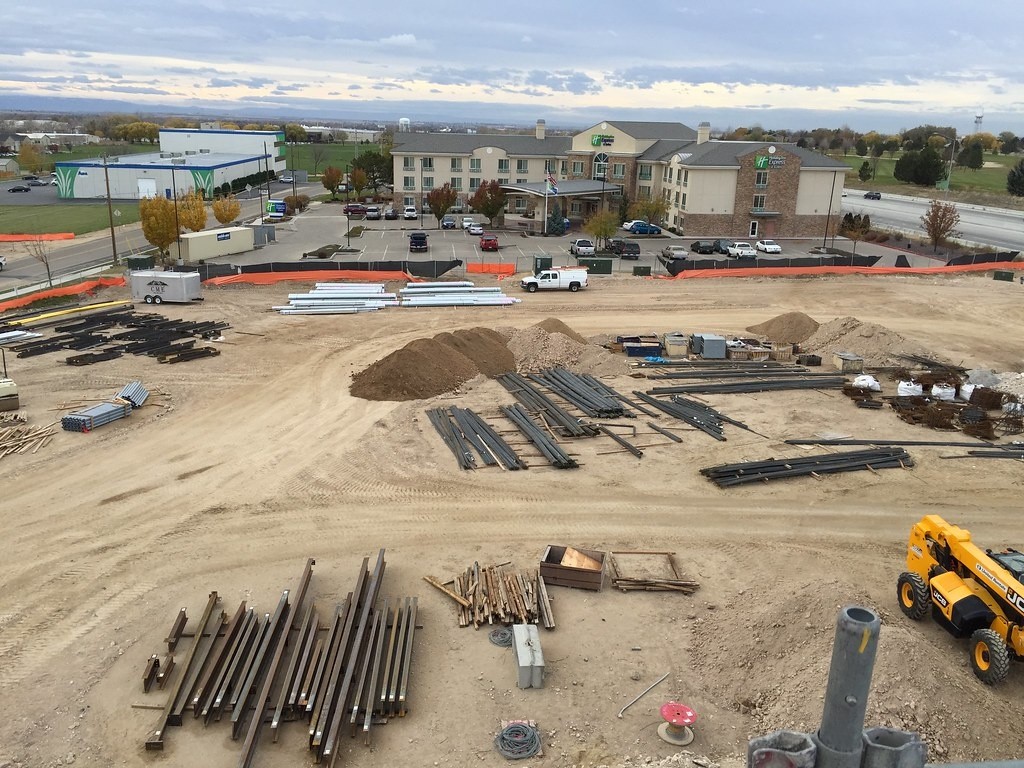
[
  {"x1": 403, "y1": 206, "x2": 418, "y2": 220},
  {"x1": 408, "y1": 232, "x2": 430, "y2": 252},
  {"x1": 441, "y1": 216, "x2": 456, "y2": 229},
  {"x1": 479, "y1": 232, "x2": 498, "y2": 251},
  {"x1": 7, "y1": 185, "x2": 32, "y2": 193},
  {"x1": 755, "y1": 239, "x2": 783, "y2": 254},
  {"x1": 662, "y1": 245, "x2": 690, "y2": 260},
  {"x1": 278, "y1": 177, "x2": 294, "y2": 184},
  {"x1": 385, "y1": 208, "x2": 399, "y2": 220},
  {"x1": 864, "y1": 191, "x2": 881, "y2": 200},
  {"x1": 365, "y1": 207, "x2": 382, "y2": 220},
  {"x1": 623, "y1": 220, "x2": 661, "y2": 235},
  {"x1": 21, "y1": 171, "x2": 59, "y2": 186},
  {"x1": 714, "y1": 239, "x2": 734, "y2": 254},
  {"x1": 343, "y1": 204, "x2": 367, "y2": 216},
  {"x1": 467, "y1": 222, "x2": 484, "y2": 235},
  {"x1": 461, "y1": 218, "x2": 474, "y2": 229},
  {"x1": 691, "y1": 240, "x2": 714, "y2": 254}
]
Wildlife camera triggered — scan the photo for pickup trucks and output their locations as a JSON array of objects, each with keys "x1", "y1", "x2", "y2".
[
  {"x1": 520, "y1": 266, "x2": 588, "y2": 292},
  {"x1": 570, "y1": 239, "x2": 596, "y2": 258},
  {"x1": 726, "y1": 242, "x2": 758, "y2": 260}
]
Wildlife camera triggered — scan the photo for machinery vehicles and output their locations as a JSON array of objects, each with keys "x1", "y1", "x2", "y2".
[{"x1": 896, "y1": 515, "x2": 1024, "y2": 687}]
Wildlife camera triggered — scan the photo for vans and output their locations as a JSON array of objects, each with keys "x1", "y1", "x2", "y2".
[{"x1": 606, "y1": 238, "x2": 640, "y2": 261}]
